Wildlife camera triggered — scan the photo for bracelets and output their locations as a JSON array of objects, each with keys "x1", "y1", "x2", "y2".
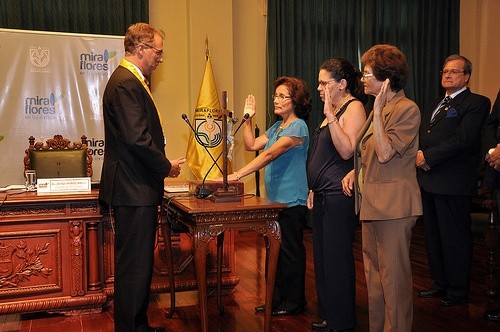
[
  {"x1": 327, "y1": 117, "x2": 337, "y2": 124},
  {"x1": 234, "y1": 171, "x2": 240, "y2": 181}
]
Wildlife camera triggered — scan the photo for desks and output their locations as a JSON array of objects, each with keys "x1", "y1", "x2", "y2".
[
  {"x1": 159, "y1": 188, "x2": 288, "y2": 332},
  {"x1": 0, "y1": 187, "x2": 240, "y2": 316}
]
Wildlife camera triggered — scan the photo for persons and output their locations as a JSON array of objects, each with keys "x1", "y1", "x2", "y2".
[
  {"x1": 98, "y1": 23, "x2": 186, "y2": 332},
  {"x1": 340, "y1": 44, "x2": 427, "y2": 332},
  {"x1": 305, "y1": 58, "x2": 368, "y2": 332},
  {"x1": 214, "y1": 77, "x2": 311, "y2": 315},
  {"x1": 414, "y1": 54, "x2": 492, "y2": 306},
  {"x1": 479, "y1": 85, "x2": 500, "y2": 320}
]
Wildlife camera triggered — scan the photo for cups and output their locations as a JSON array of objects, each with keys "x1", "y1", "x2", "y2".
[{"x1": 25, "y1": 170, "x2": 36, "y2": 188}]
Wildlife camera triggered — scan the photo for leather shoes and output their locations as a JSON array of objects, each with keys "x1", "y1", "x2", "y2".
[
  {"x1": 272, "y1": 305, "x2": 306, "y2": 316},
  {"x1": 416, "y1": 284, "x2": 447, "y2": 297},
  {"x1": 255, "y1": 306, "x2": 266, "y2": 312},
  {"x1": 311, "y1": 320, "x2": 328, "y2": 330},
  {"x1": 440, "y1": 294, "x2": 469, "y2": 307},
  {"x1": 484, "y1": 308, "x2": 500, "y2": 321}
]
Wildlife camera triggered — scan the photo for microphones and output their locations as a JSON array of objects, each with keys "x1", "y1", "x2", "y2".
[
  {"x1": 181, "y1": 113, "x2": 236, "y2": 195},
  {"x1": 194, "y1": 113, "x2": 249, "y2": 198}
]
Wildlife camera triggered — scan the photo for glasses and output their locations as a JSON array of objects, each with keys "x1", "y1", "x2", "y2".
[
  {"x1": 361, "y1": 72, "x2": 373, "y2": 78},
  {"x1": 440, "y1": 68, "x2": 465, "y2": 74},
  {"x1": 318, "y1": 80, "x2": 335, "y2": 86},
  {"x1": 271, "y1": 93, "x2": 292, "y2": 101},
  {"x1": 141, "y1": 42, "x2": 164, "y2": 55}
]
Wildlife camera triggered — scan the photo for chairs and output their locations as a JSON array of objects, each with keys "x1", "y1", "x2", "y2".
[
  {"x1": 24, "y1": 133, "x2": 94, "y2": 178},
  {"x1": 466, "y1": 162, "x2": 500, "y2": 312}
]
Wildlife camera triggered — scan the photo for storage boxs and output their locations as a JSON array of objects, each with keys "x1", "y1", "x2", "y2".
[{"x1": 191, "y1": 181, "x2": 245, "y2": 195}]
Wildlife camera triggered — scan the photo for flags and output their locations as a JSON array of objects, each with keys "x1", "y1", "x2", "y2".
[{"x1": 185, "y1": 55, "x2": 235, "y2": 182}]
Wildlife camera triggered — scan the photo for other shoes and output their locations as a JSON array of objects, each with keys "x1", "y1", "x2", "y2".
[{"x1": 149, "y1": 326, "x2": 165, "y2": 332}]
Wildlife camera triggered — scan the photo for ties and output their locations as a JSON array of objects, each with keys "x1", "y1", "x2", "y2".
[
  {"x1": 144, "y1": 77, "x2": 151, "y2": 92},
  {"x1": 431, "y1": 96, "x2": 450, "y2": 123}
]
